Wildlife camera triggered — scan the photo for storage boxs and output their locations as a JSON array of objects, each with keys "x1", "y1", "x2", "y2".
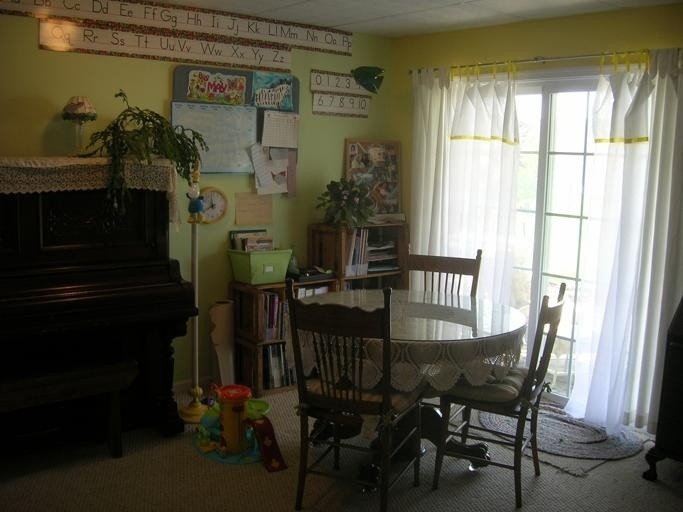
[{"x1": 227, "y1": 247, "x2": 293, "y2": 285}]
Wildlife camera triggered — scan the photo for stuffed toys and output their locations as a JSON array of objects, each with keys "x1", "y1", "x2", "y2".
[{"x1": 186, "y1": 160, "x2": 210, "y2": 225}]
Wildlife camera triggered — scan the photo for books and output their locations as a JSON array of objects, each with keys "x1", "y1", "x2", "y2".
[
  {"x1": 312, "y1": 225, "x2": 400, "y2": 276},
  {"x1": 233, "y1": 285, "x2": 331, "y2": 389},
  {"x1": 227, "y1": 227, "x2": 277, "y2": 252}
]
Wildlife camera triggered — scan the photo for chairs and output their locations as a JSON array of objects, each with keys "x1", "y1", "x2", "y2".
[
  {"x1": 285, "y1": 280, "x2": 426, "y2": 512},
  {"x1": 430, "y1": 283, "x2": 568, "y2": 508},
  {"x1": 408, "y1": 249, "x2": 482, "y2": 408}
]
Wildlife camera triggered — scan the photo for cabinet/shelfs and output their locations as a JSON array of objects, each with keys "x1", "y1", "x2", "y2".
[
  {"x1": 308, "y1": 221, "x2": 409, "y2": 291},
  {"x1": 0, "y1": 157, "x2": 199, "y2": 452},
  {"x1": 234, "y1": 281, "x2": 339, "y2": 397}
]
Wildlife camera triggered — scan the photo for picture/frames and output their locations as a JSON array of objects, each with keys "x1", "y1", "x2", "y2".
[{"x1": 345, "y1": 137, "x2": 403, "y2": 220}]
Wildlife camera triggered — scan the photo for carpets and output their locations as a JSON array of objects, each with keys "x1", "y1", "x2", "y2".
[
  {"x1": 479, "y1": 394, "x2": 644, "y2": 461},
  {"x1": 449, "y1": 402, "x2": 650, "y2": 478}
]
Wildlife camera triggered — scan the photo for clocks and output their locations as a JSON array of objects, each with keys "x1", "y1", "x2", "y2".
[{"x1": 197, "y1": 186, "x2": 228, "y2": 224}]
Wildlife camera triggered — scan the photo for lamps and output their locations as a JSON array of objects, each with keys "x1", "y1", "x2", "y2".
[{"x1": 62, "y1": 95, "x2": 98, "y2": 158}]
